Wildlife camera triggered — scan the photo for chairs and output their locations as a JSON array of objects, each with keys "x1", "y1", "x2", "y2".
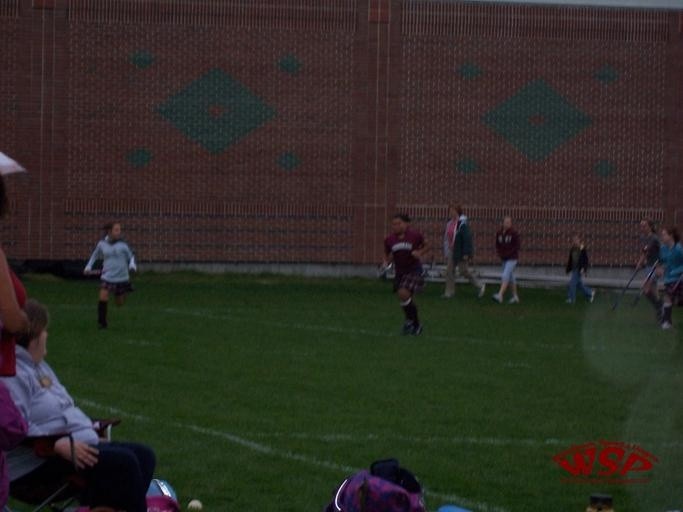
[{"x1": 0, "y1": 382, "x2": 121, "y2": 511}]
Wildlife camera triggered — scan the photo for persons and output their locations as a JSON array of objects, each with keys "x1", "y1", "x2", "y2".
[
  {"x1": 438, "y1": 204, "x2": 488, "y2": 297},
  {"x1": 653, "y1": 225, "x2": 683, "y2": 329},
  {"x1": 380, "y1": 213, "x2": 430, "y2": 335},
  {"x1": 0, "y1": 297, "x2": 159, "y2": 511},
  {"x1": 638, "y1": 217, "x2": 666, "y2": 323},
  {"x1": 84, "y1": 220, "x2": 138, "y2": 332},
  {"x1": 1, "y1": 172, "x2": 33, "y2": 384},
  {"x1": 564, "y1": 233, "x2": 596, "y2": 305},
  {"x1": 491, "y1": 214, "x2": 523, "y2": 304}
]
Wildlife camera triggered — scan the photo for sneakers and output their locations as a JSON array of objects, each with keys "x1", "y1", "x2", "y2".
[
  {"x1": 561, "y1": 288, "x2": 595, "y2": 304},
  {"x1": 96, "y1": 320, "x2": 108, "y2": 330},
  {"x1": 439, "y1": 280, "x2": 519, "y2": 305},
  {"x1": 399, "y1": 319, "x2": 423, "y2": 336},
  {"x1": 655, "y1": 296, "x2": 673, "y2": 330}
]
